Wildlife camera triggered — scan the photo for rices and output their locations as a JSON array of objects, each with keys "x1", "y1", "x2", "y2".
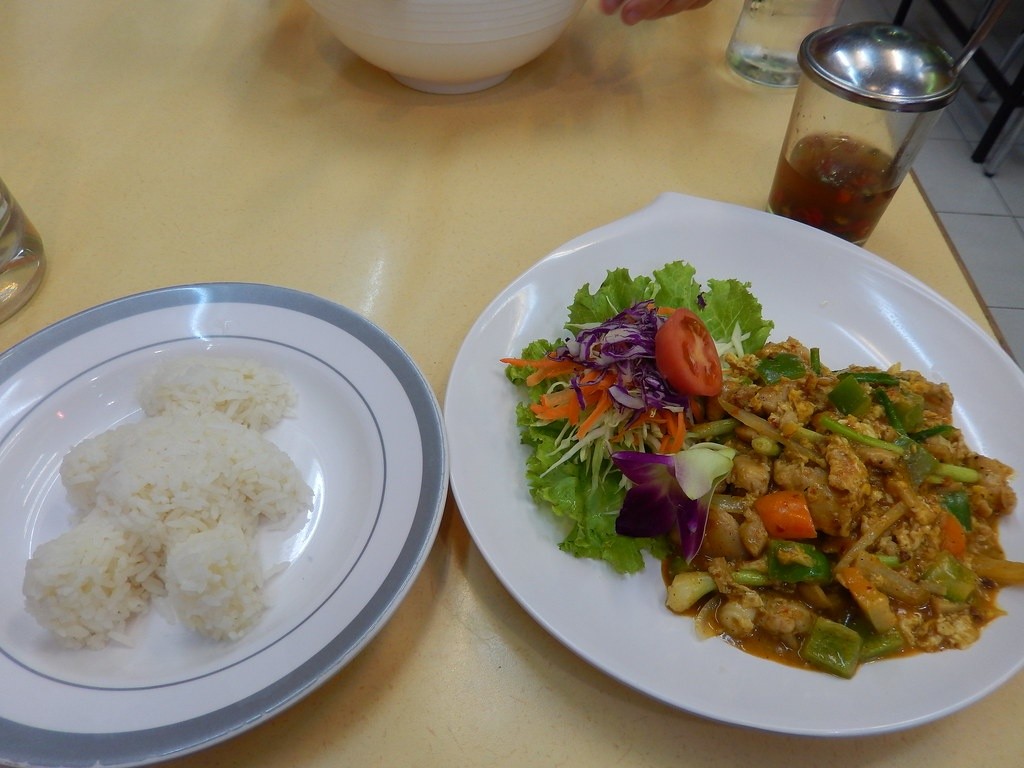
[{"x1": 22, "y1": 353, "x2": 316, "y2": 649}]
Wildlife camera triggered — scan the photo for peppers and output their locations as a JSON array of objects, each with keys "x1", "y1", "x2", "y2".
[{"x1": 745, "y1": 353, "x2": 1004, "y2": 679}]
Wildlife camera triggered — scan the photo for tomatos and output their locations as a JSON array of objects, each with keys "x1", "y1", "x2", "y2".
[{"x1": 658, "y1": 308, "x2": 723, "y2": 397}]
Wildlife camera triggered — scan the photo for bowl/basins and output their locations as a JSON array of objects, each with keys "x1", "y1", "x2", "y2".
[{"x1": 304, "y1": 0, "x2": 585, "y2": 94}]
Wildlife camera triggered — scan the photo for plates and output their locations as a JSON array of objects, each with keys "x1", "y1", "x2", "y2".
[
  {"x1": 0, "y1": 282, "x2": 450, "y2": 768},
  {"x1": 443, "y1": 192, "x2": 1024, "y2": 738}
]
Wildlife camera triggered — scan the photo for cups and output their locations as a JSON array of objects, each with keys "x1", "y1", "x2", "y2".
[
  {"x1": 765, "y1": 21, "x2": 964, "y2": 246},
  {"x1": 727, "y1": 0, "x2": 843, "y2": 88},
  {"x1": 0, "y1": 177, "x2": 45, "y2": 324}
]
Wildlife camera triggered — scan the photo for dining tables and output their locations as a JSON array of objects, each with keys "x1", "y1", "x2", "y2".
[{"x1": 1, "y1": 1, "x2": 1022, "y2": 768}]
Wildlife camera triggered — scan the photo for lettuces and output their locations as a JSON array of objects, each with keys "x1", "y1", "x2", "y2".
[{"x1": 496, "y1": 260, "x2": 774, "y2": 575}]
